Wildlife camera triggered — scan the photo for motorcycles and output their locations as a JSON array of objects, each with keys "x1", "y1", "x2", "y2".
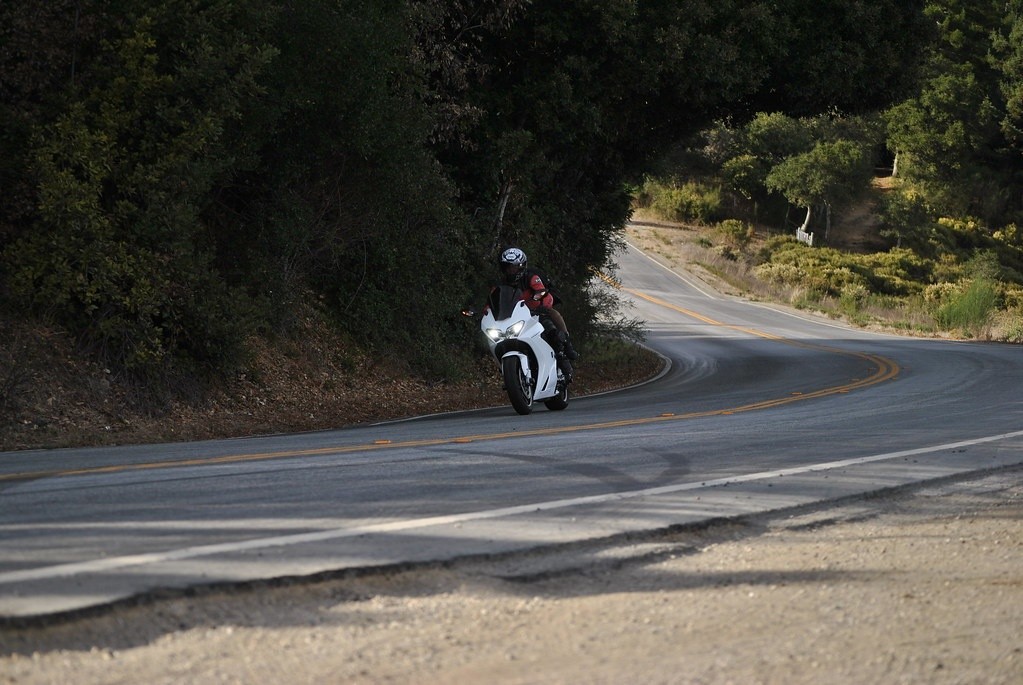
[{"x1": 460, "y1": 278, "x2": 570, "y2": 414}]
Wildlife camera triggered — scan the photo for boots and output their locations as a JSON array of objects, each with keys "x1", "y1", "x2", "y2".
[
  {"x1": 554, "y1": 351, "x2": 574, "y2": 384},
  {"x1": 562, "y1": 331, "x2": 581, "y2": 360},
  {"x1": 502, "y1": 383, "x2": 506, "y2": 390}
]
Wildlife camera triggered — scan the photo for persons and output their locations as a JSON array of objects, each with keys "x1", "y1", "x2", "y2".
[{"x1": 482, "y1": 248, "x2": 580, "y2": 361}]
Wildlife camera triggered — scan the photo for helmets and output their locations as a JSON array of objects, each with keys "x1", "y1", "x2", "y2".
[{"x1": 500, "y1": 249, "x2": 527, "y2": 281}]
